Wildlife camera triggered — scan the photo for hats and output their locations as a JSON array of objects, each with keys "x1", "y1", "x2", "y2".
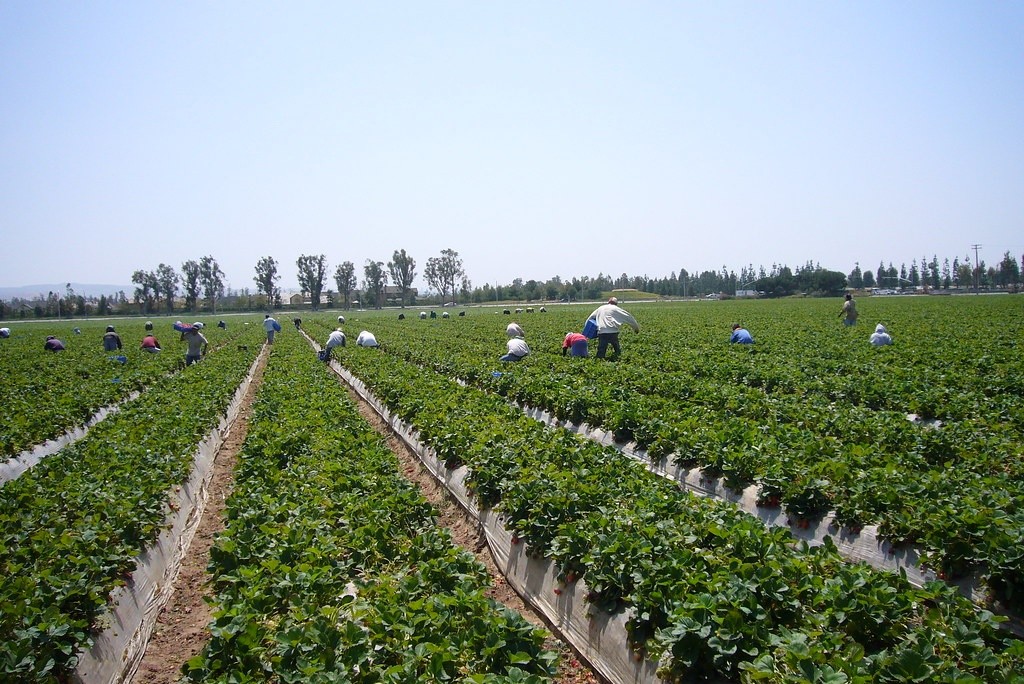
[
  {"x1": 609, "y1": 296, "x2": 618, "y2": 304},
  {"x1": 192, "y1": 325, "x2": 199, "y2": 330}
]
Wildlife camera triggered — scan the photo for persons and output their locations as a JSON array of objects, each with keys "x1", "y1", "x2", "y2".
[
  {"x1": 526, "y1": 307, "x2": 534, "y2": 313},
  {"x1": 443, "y1": 312, "x2": 449, "y2": 318},
  {"x1": 0, "y1": 328, "x2": 10, "y2": 338},
  {"x1": 838, "y1": 295, "x2": 857, "y2": 326},
  {"x1": 103, "y1": 325, "x2": 122, "y2": 351},
  {"x1": 141, "y1": 333, "x2": 161, "y2": 353},
  {"x1": 459, "y1": 311, "x2": 465, "y2": 316},
  {"x1": 562, "y1": 332, "x2": 588, "y2": 357},
  {"x1": 585, "y1": 297, "x2": 639, "y2": 358},
  {"x1": 506, "y1": 322, "x2": 525, "y2": 336},
  {"x1": 516, "y1": 308, "x2": 523, "y2": 313},
  {"x1": 356, "y1": 331, "x2": 378, "y2": 347},
  {"x1": 338, "y1": 316, "x2": 345, "y2": 323},
  {"x1": 430, "y1": 311, "x2": 436, "y2": 318},
  {"x1": 294, "y1": 318, "x2": 301, "y2": 330},
  {"x1": 181, "y1": 321, "x2": 208, "y2": 366},
  {"x1": 398, "y1": 314, "x2": 405, "y2": 320},
  {"x1": 145, "y1": 321, "x2": 153, "y2": 331},
  {"x1": 870, "y1": 324, "x2": 892, "y2": 345},
  {"x1": 731, "y1": 323, "x2": 752, "y2": 344},
  {"x1": 263, "y1": 315, "x2": 276, "y2": 345},
  {"x1": 74, "y1": 327, "x2": 81, "y2": 334},
  {"x1": 500, "y1": 336, "x2": 530, "y2": 361},
  {"x1": 420, "y1": 312, "x2": 426, "y2": 319},
  {"x1": 326, "y1": 328, "x2": 346, "y2": 364},
  {"x1": 217, "y1": 321, "x2": 226, "y2": 330},
  {"x1": 503, "y1": 309, "x2": 510, "y2": 314},
  {"x1": 540, "y1": 306, "x2": 546, "y2": 312},
  {"x1": 44, "y1": 336, "x2": 64, "y2": 352}
]
